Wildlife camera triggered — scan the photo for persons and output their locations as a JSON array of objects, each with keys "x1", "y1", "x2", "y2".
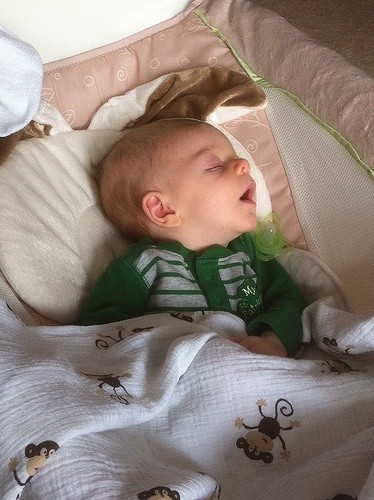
[{"x1": 81, "y1": 119, "x2": 313, "y2": 500}]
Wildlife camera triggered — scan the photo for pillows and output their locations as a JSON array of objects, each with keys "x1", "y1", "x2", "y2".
[{"x1": 0, "y1": 119, "x2": 274, "y2": 323}]
